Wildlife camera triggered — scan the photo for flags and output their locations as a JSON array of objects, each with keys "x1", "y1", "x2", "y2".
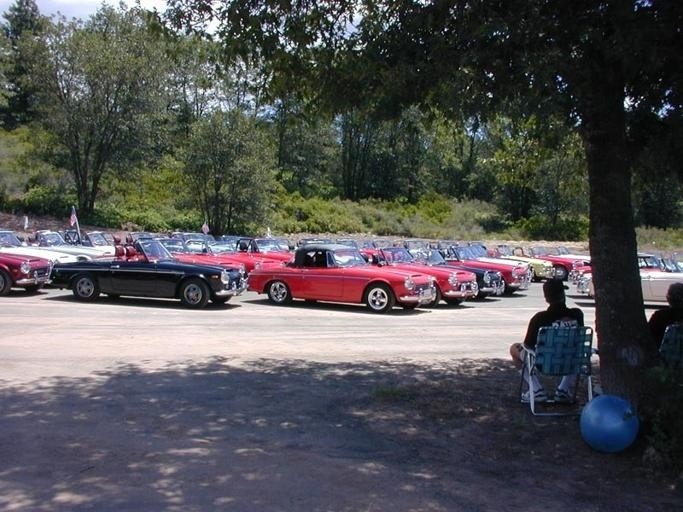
[
  {"x1": 201, "y1": 221, "x2": 209, "y2": 235},
  {"x1": 69, "y1": 208, "x2": 76, "y2": 226}
]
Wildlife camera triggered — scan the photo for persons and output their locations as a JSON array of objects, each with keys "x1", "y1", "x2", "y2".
[
  {"x1": 643, "y1": 282, "x2": 683, "y2": 350},
  {"x1": 509, "y1": 278, "x2": 584, "y2": 402}
]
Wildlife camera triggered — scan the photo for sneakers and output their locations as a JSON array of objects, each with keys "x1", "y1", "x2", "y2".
[
  {"x1": 554, "y1": 387, "x2": 574, "y2": 403},
  {"x1": 522, "y1": 388, "x2": 547, "y2": 402}
]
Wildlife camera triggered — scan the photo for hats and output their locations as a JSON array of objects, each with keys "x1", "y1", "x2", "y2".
[{"x1": 543, "y1": 278, "x2": 568, "y2": 297}]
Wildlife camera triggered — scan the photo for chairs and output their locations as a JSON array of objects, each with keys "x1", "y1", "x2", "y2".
[
  {"x1": 112, "y1": 232, "x2": 139, "y2": 263},
  {"x1": 515, "y1": 323, "x2": 596, "y2": 419},
  {"x1": 238, "y1": 241, "x2": 248, "y2": 252}
]
